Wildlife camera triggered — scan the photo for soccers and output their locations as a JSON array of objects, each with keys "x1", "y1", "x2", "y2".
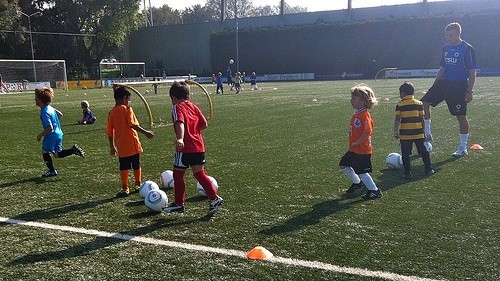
[
  {"x1": 218, "y1": 90, "x2": 221, "y2": 93},
  {"x1": 160, "y1": 170, "x2": 173, "y2": 188},
  {"x1": 139, "y1": 181, "x2": 159, "y2": 198},
  {"x1": 145, "y1": 90, "x2": 149, "y2": 93},
  {"x1": 144, "y1": 188, "x2": 169, "y2": 213},
  {"x1": 386, "y1": 152, "x2": 403, "y2": 169},
  {"x1": 230, "y1": 59, "x2": 234, "y2": 64},
  {"x1": 197, "y1": 176, "x2": 219, "y2": 196}
]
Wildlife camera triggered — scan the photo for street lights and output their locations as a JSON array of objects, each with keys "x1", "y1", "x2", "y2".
[{"x1": 17, "y1": 10, "x2": 43, "y2": 82}]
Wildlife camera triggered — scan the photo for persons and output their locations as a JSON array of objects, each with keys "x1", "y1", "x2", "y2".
[
  {"x1": 420, "y1": 22, "x2": 476, "y2": 158},
  {"x1": 151, "y1": 76, "x2": 159, "y2": 93},
  {"x1": 35, "y1": 87, "x2": 87, "y2": 177},
  {"x1": 211, "y1": 73, "x2": 216, "y2": 84},
  {"x1": 250, "y1": 72, "x2": 261, "y2": 91},
  {"x1": 226, "y1": 66, "x2": 246, "y2": 94},
  {"x1": 105, "y1": 85, "x2": 156, "y2": 198},
  {"x1": 394, "y1": 81, "x2": 435, "y2": 180},
  {"x1": 216, "y1": 72, "x2": 223, "y2": 94},
  {"x1": 338, "y1": 83, "x2": 384, "y2": 200},
  {"x1": 163, "y1": 81, "x2": 224, "y2": 217},
  {"x1": 77, "y1": 100, "x2": 97, "y2": 125}
]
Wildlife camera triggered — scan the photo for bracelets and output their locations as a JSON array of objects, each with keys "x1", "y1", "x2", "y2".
[{"x1": 466, "y1": 90, "x2": 474, "y2": 95}]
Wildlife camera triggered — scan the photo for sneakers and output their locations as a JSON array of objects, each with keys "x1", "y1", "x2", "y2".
[
  {"x1": 207, "y1": 195, "x2": 224, "y2": 214},
  {"x1": 117, "y1": 188, "x2": 130, "y2": 197},
  {"x1": 362, "y1": 188, "x2": 383, "y2": 201},
  {"x1": 425, "y1": 169, "x2": 435, "y2": 176},
  {"x1": 42, "y1": 170, "x2": 57, "y2": 177},
  {"x1": 134, "y1": 185, "x2": 141, "y2": 192},
  {"x1": 72, "y1": 145, "x2": 84, "y2": 158},
  {"x1": 346, "y1": 179, "x2": 365, "y2": 193},
  {"x1": 402, "y1": 171, "x2": 412, "y2": 179},
  {"x1": 163, "y1": 202, "x2": 185, "y2": 213},
  {"x1": 424, "y1": 134, "x2": 433, "y2": 141},
  {"x1": 78, "y1": 120, "x2": 84, "y2": 125},
  {"x1": 452, "y1": 149, "x2": 469, "y2": 157}
]
